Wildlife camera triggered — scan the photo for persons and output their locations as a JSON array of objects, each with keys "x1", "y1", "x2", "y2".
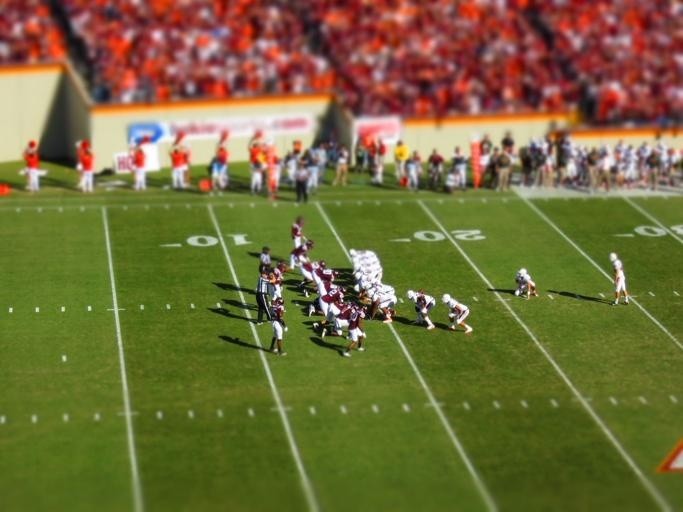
[
  {"x1": 0, "y1": 0, "x2": 683, "y2": 204},
  {"x1": 512, "y1": 268, "x2": 538, "y2": 300},
  {"x1": 256, "y1": 216, "x2": 473, "y2": 358},
  {"x1": 609, "y1": 252, "x2": 629, "y2": 306}
]
